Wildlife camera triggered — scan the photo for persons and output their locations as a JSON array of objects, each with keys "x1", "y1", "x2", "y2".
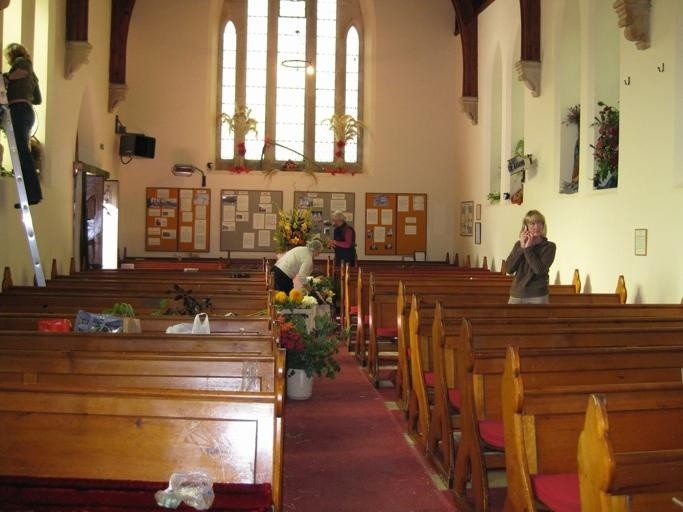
[
  {"x1": 273, "y1": 240, "x2": 322, "y2": 297},
  {"x1": 327, "y1": 210, "x2": 355, "y2": 267},
  {"x1": 506, "y1": 210, "x2": 556, "y2": 304},
  {"x1": 3, "y1": 43, "x2": 43, "y2": 208}
]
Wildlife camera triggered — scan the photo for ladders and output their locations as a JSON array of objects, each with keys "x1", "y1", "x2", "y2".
[{"x1": 2, "y1": 108, "x2": 46, "y2": 287}]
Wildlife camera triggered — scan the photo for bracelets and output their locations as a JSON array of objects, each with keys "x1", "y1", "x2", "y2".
[{"x1": 6, "y1": 75, "x2": 11, "y2": 81}]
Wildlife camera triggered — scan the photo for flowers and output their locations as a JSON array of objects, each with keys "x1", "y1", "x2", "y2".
[
  {"x1": 275, "y1": 287, "x2": 318, "y2": 313},
  {"x1": 274, "y1": 310, "x2": 354, "y2": 380},
  {"x1": 588, "y1": 101, "x2": 619, "y2": 189},
  {"x1": 270, "y1": 200, "x2": 316, "y2": 249},
  {"x1": 226, "y1": 141, "x2": 254, "y2": 175},
  {"x1": 318, "y1": 139, "x2": 355, "y2": 176}
]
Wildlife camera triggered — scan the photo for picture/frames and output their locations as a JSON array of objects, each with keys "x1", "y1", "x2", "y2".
[
  {"x1": 475, "y1": 222, "x2": 481, "y2": 244},
  {"x1": 476, "y1": 204, "x2": 481, "y2": 220},
  {"x1": 634, "y1": 229, "x2": 647, "y2": 256}
]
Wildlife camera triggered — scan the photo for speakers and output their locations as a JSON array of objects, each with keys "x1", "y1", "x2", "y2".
[
  {"x1": 144, "y1": 136, "x2": 156, "y2": 158},
  {"x1": 120, "y1": 133, "x2": 145, "y2": 159}
]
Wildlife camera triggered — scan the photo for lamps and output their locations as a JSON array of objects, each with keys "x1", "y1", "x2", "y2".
[
  {"x1": 281, "y1": 29, "x2": 315, "y2": 76},
  {"x1": 171, "y1": 163, "x2": 206, "y2": 187}
]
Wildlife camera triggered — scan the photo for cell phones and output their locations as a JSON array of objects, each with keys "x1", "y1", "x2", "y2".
[{"x1": 524, "y1": 226, "x2": 528, "y2": 232}]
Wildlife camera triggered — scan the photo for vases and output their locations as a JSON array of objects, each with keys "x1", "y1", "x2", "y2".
[{"x1": 287, "y1": 368, "x2": 313, "y2": 400}]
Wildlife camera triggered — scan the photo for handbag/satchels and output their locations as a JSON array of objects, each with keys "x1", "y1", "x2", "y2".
[
  {"x1": 73, "y1": 302, "x2": 142, "y2": 334},
  {"x1": 35, "y1": 317, "x2": 71, "y2": 333}
]
